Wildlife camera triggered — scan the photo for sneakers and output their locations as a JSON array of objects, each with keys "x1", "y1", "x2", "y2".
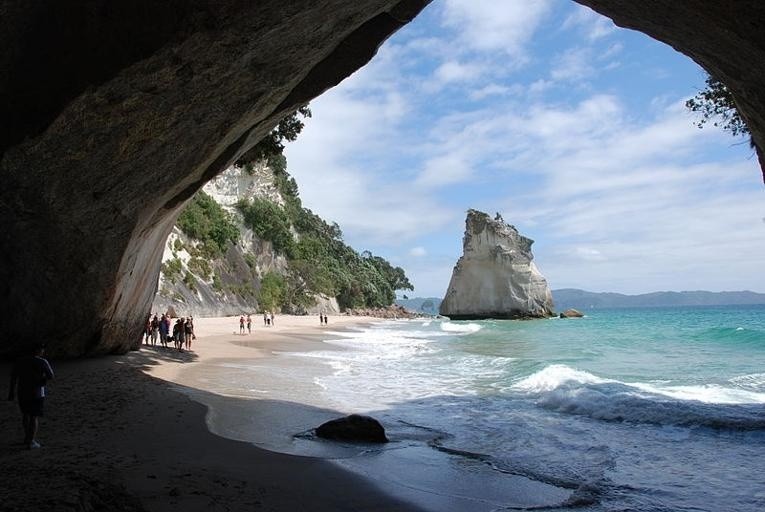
[{"x1": 30, "y1": 439, "x2": 40, "y2": 449}]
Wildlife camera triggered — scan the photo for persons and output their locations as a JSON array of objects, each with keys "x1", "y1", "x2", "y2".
[
  {"x1": 8, "y1": 341, "x2": 56, "y2": 450},
  {"x1": 264, "y1": 310, "x2": 274, "y2": 326},
  {"x1": 320, "y1": 313, "x2": 323, "y2": 325},
  {"x1": 324, "y1": 315, "x2": 327, "y2": 324},
  {"x1": 145, "y1": 314, "x2": 197, "y2": 353},
  {"x1": 239, "y1": 315, "x2": 252, "y2": 335}
]
